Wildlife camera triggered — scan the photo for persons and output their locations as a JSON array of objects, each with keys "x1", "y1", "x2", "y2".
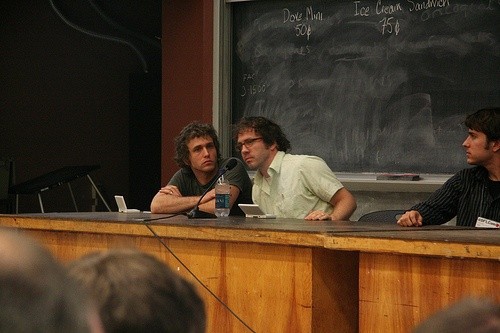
[
  {"x1": 231, "y1": 116, "x2": 358, "y2": 221},
  {"x1": 0, "y1": 230, "x2": 208, "y2": 333},
  {"x1": 151, "y1": 125, "x2": 253, "y2": 217},
  {"x1": 397, "y1": 108, "x2": 500, "y2": 227},
  {"x1": 409, "y1": 291, "x2": 500, "y2": 333}
]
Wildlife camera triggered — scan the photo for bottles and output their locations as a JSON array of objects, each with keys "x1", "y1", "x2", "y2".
[{"x1": 214, "y1": 169, "x2": 233, "y2": 217}]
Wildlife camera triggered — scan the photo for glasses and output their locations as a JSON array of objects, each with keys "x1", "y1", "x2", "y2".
[{"x1": 235, "y1": 138, "x2": 262, "y2": 152}]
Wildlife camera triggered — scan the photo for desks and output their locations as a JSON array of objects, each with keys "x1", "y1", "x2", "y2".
[{"x1": 0, "y1": 212, "x2": 500, "y2": 333}]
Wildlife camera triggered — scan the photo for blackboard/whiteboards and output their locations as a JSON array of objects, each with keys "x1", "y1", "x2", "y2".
[{"x1": 213, "y1": 0, "x2": 500, "y2": 193}]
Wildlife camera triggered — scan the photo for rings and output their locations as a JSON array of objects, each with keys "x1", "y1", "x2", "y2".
[
  {"x1": 170, "y1": 192, "x2": 173, "y2": 195},
  {"x1": 320, "y1": 213, "x2": 324, "y2": 216}
]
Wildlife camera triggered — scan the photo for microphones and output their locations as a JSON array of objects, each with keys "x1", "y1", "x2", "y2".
[{"x1": 187, "y1": 159, "x2": 238, "y2": 219}]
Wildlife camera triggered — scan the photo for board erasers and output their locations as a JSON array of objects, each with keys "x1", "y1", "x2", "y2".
[{"x1": 375, "y1": 174, "x2": 420, "y2": 181}]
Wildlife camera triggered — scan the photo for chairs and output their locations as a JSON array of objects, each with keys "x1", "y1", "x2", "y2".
[{"x1": 358, "y1": 210, "x2": 412, "y2": 223}]
